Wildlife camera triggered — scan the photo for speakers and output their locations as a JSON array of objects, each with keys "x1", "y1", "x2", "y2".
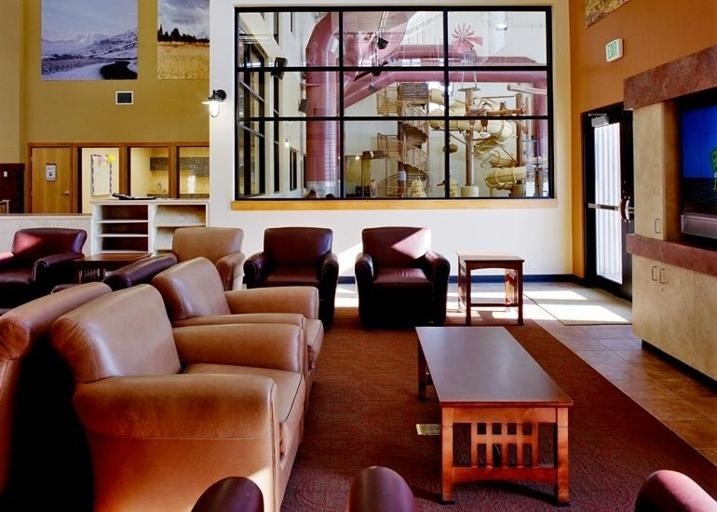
[
  {"x1": 298, "y1": 99, "x2": 308, "y2": 112},
  {"x1": 270, "y1": 57, "x2": 288, "y2": 81}
]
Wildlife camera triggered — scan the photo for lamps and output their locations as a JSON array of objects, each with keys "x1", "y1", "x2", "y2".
[{"x1": 200, "y1": 89, "x2": 227, "y2": 119}]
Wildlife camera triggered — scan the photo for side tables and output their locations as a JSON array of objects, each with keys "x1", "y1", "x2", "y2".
[{"x1": 455, "y1": 253, "x2": 526, "y2": 326}]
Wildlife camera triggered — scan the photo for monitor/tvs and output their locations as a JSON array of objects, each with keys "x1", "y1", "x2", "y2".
[{"x1": 676, "y1": 87, "x2": 717, "y2": 251}]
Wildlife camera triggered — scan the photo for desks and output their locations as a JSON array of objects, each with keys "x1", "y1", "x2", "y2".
[{"x1": 411, "y1": 327, "x2": 574, "y2": 503}]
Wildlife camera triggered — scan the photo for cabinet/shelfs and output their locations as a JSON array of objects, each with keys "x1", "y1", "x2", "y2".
[
  {"x1": 630, "y1": 254, "x2": 716, "y2": 380},
  {"x1": 632, "y1": 103, "x2": 679, "y2": 240},
  {"x1": 87, "y1": 200, "x2": 208, "y2": 255},
  {"x1": 0, "y1": 212, "x2": 91, "y2": 256}
]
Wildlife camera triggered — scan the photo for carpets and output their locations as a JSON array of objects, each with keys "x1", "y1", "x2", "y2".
[
  {"x1": 280, "y1": 303, "x2": 716, "y2": 512},
  {"x1": 521, "y1": 288, "x2": 634, "y2": 326}
]
